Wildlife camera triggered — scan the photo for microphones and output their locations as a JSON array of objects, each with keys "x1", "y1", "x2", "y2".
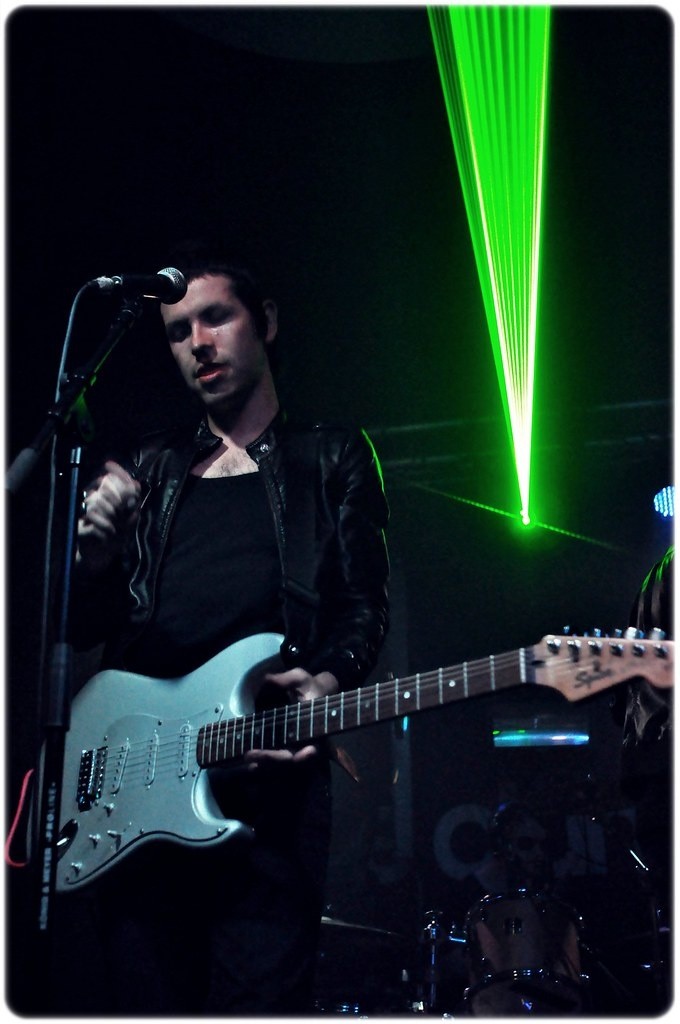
[{"x1": 96, "y1": 266, "x2": 187, "y2": 304}]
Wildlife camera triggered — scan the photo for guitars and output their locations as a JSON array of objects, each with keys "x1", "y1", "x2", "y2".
[{"x1": 25, "y1": 628, "x2": 673, "y2": 897}]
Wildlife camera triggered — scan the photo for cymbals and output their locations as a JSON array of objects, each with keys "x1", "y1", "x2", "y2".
[{"x1": 322, "y1": 910, "x2": 398, "y2": 937}]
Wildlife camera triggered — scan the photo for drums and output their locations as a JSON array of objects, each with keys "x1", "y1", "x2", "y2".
[
  {"x1": 454, "y1": 886, "x2": 591, "y2": 1015},
  {"x1": 316, "y1": 997, "x2": 385, "y2": 1013}
]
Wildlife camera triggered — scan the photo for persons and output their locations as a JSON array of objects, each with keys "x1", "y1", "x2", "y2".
[
  {"x1": 461, "y1": 799, "x2": 581, "y2": 975},
  {"x1": 53, "y1": 263, "x2": 391, "y2": 1014},
  {"x1": 607, "y1": 480, "x2": 673, "y2": 940}
]
